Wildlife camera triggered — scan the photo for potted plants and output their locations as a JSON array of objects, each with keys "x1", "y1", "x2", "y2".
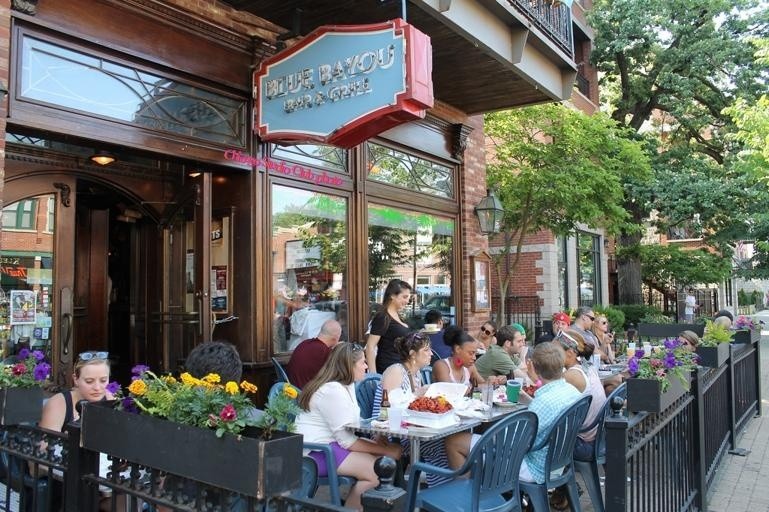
[{"x1": 697, "y1": 319, "x2": 734, "y2": 369}]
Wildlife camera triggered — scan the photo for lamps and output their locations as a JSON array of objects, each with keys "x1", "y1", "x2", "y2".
[{"x1": 474, "y1": 196, "x2": 505, "y2": 237}]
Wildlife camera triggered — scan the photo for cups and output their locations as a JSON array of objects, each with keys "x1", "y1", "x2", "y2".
[
  {"x1": 644, "y1": 342, "x2": 650, "y2": 346},
  {"x1": 387, "y1": 408, "x2": 402, "y2": 433},
  {"x1": 643, "y1": 344, "x2": 651, "y2": 357},
  {"x1": 628, "y1": 348, "x2": 635, "y2": 357},
  {"x1": 506, "y1": 379, "x2": 521, "y2": 402},
  {"x1": 482, "y1": 384, "x2": 493, "y2": 413},
  {"x1": 629, "y1": 343, "x2": 635, "y2": 348}
]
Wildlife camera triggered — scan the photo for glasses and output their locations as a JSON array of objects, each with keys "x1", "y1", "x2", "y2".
[
  {"x1": 585, "y1": 312, "x2": 596, "y2": 323},
  {"x1": 556, "y1": 329, "x2": 579, "y2": 346},
  {"x1": 412, "y1": 334, "x2": 427, "y2": 344},
  {"x1": 600, "y1": 322, "x2": 612, "y2": 326},
  {"x1": 351, "y1": 341, "x2": 366, "y2": 351},
  {"x1": 690, "y1": 292, "x2": 694, "y2": 294},
  {"x1": 79, "y1": 351, "x2": 109, "y2": 361},
  {"x1": 480, "y1": 325, "x2": 492, "y2": 338}
]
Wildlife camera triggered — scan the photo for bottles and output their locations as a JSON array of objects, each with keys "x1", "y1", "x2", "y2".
[
  {"x1": 471, "y1": 379, "x2": 481, "y2": 401},
  {"x1": 509, "y1": 370, "x2": 514, "y2": 380},
  {"x1": 380, "y1": 389, "x2": 390, "y2": 418}
]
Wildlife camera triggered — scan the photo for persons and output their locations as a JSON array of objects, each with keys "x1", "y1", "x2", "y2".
[
  {"x1": 470, "y1": 320, "x2": 497, "y2": 350},
  {"x1": 685, "y1": 288, "x2": 704, "y2": 324},
  {"x1": 474, "y1": 325, "x2": 534, "y2": 387},
  {"x1": 417, "y1": 309, "x2": 453, "y2": 366},
  {"x1": 187, "y1": 340, "x2": 270, "y2": 430},
  {"x1": 445, "y1": 342, "x2": 582, "y2": 485},
  {"x1": 363, "y1": 280, "x2": 414, "y2": 375},
  {"x1": 370, "y1": 332, "x2": 455, "y2": 488},
  {"x1": 292, "y1": 342, "x2": 403, "y2": 512},
  {"x1": 536, "y1": 312, "x2": 570, "y2": 344},
  {"x1": 549, "y1": 330, "x2": 608, "y2": 510},
  {"x1": 286, "y1": 320, "x2": 343, "y2": 392},
  {"x1": 511, "y1": 322, "x2": 534, "y2": 369},
  {"x1": 432, "y1": 324, "x2": 487, "y2": 401},
  {"x1": 28, "y1": 350, "x2": 127, "y2": 481},
  {"x1": 592, "y1": 316, "x2": 613, "y2": 364},
  {"x1": 677, "y1": 329, "x2": 704, "y2": 366},
  {"x1": 571, "y1": 306, "x2": 608, "y2": 367}
]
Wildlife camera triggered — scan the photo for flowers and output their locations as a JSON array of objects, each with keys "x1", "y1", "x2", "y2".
[
  {"x1": 101, "y1": 362, "x2": 302, "y2": 441},
  {"x1": 0, "y1": 345, "x2": 51, "y2": 387},
  {"x1": 734, "y1": 313, "x2": 766, "y2": 332},
  {"x1": 628, "y1": 334, "x2": 701, "y2": 393}
]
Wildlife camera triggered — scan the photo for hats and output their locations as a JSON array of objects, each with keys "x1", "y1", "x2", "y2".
[
  {"x1": 550, "y1": 312, "x2": 573, "y2": 328},
  {"x1": 680, "y1": 329, "x2": 699, "y2": 345},
  {"x1": 511, "y1": 322, "x2": 525, "y2": 334}
]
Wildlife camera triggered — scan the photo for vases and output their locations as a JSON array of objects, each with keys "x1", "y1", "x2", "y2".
[
  {"x1": 733, "y1": 327, "x2": 762, "y2": 344},
  {"x1": 627, "y1": 364, "x2": 693, "y2": 414},
  {"x1": 77, "y1": 398, "x2": 305, "y2": 502},
  {"x1": 1, "y1": 390, "x2": 45, "y2": 426}
]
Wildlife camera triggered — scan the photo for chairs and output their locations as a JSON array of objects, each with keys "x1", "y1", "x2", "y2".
[
  {"x1": 419, "y1": 367, "x2": 435, "y2": 384},
  {"x1": 354, "y1": 373, "x2": 413, "y2": 486},
  {"x1": 573, "y1": 381, "x2": 628, "y2": 512},
  {"x1": 0, "y1": 397, "x2": 47, "y2": 511},
  {"x1": 482, "y1": 392, "x2": 593, "y2": 511},
  {"x1": 406, "y1": 411, "x2": 539, "y2": 512},
  {"x1": 229, "y1": 455, "x2": 319, "y2": 512},
  {"x1": 267, "y1": 381, "x2": 302, "y2": 433},
  {"x1": 271, "y1": 357, "x2": 289, "y2": 382}
]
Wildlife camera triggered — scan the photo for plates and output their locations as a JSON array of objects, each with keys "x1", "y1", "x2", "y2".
[{"x1": 493, "y1": 393, "x2": 516, "y2": 407}]
